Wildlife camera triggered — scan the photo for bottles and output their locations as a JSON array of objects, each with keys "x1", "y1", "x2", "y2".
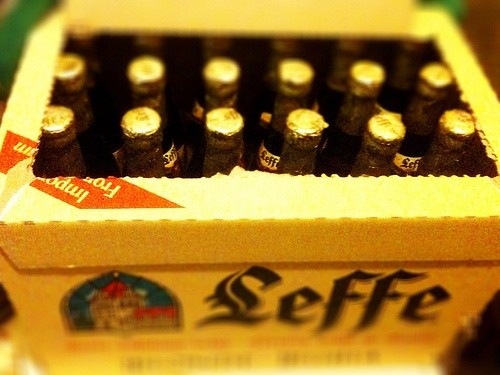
[{"x1": 36, "y1": 26, "x2": 485, "y2": 174}]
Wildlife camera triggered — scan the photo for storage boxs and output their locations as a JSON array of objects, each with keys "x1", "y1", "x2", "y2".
[{"x1": 0, "y1": 0, "x2": 500, "y2": 375}]
